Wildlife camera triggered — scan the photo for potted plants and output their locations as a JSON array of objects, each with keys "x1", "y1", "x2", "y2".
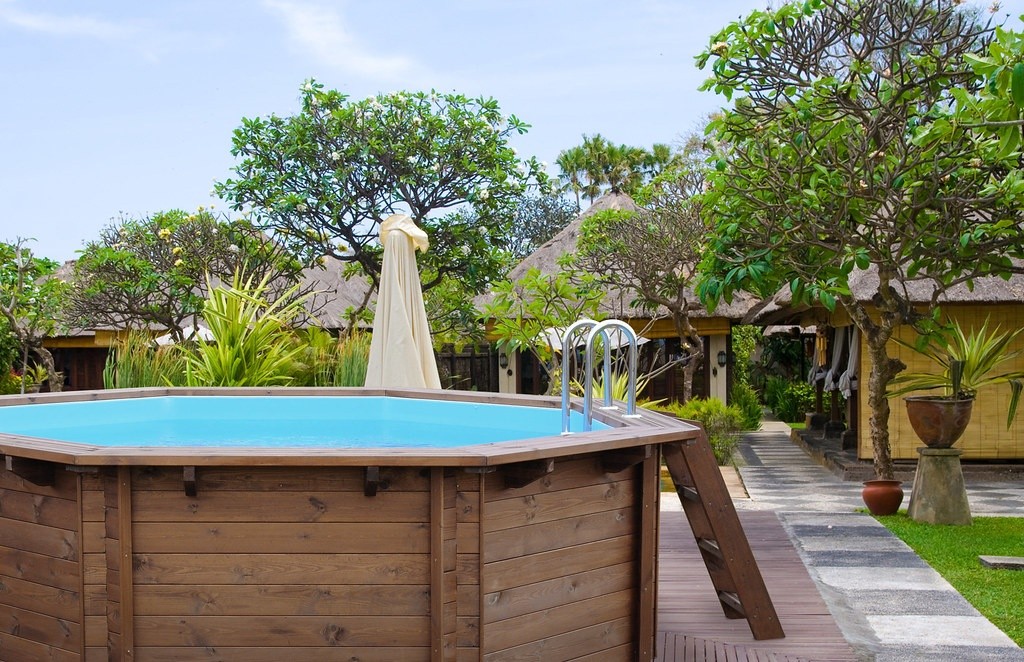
[{"x1": 888, "y1": 315, "x2": 1024, "y2": 447}]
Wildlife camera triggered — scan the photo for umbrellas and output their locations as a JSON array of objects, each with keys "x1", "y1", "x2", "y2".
[
  {"x1": 143, "y1": 323, "x2": 215, "y2": 348},
  {"x1": 525, "y1": 323, "x2": 648, "y2": 352},
  {"x1": 363, "y1": 210, "x2": 442, "y2": 390}
]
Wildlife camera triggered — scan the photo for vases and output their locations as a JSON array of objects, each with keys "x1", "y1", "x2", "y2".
[{"x1": 862, "y1": 479, "x2": 904, "y2": 515}]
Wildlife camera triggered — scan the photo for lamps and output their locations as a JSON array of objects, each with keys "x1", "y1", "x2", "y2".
[
  {"x1": 500, "y1": 353, "x2": 508, "y2": 368},
  {"x1": 717, "y1": 351, "x2": 725, "y2": 368}
]
